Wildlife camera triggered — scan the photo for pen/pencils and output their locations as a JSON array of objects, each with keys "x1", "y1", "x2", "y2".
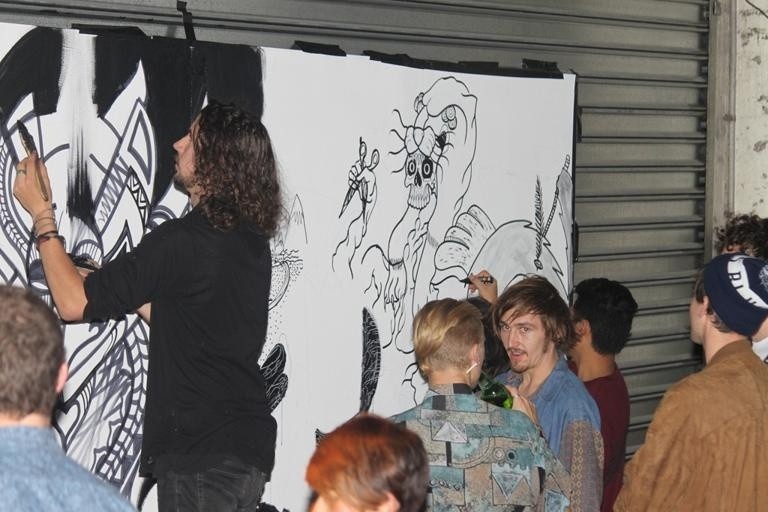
[
  {"x1": 460, "y1": 278, "x2": 493, "y2": 283},
  {"x1": 17, "y1": 120, "x2": 48, "y2": 201}
]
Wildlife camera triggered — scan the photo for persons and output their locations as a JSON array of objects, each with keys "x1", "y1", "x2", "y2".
[
  {"x1": 382, "y1": 297, "x2": 570, "y2": 512},
  {"x1": 1, "y1": 282, "x2": 136, "y2": 511},
  {"x1": 478, "y1": 272, "x2": 604, "y2": 510},
  {"x1": 465, "y1": 267, "x2": 512, "y2": 383},
  {"x1": 11, "y1": 99, "x2": 280, "y2": 510},
  {"x1": 611, "y1": 251, "x2": 768, "y2": 512},
  {"x1": 712, "y1": 210, "x2": 768, "y2": 366},
  {"x1": 565, "y1": 276, "x2": 639, "y2": 512},
  {"x1": 304, "y1": 411, "x2": 428, "y2": 511}
]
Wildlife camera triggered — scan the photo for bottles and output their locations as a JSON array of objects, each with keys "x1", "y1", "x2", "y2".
[{"x1": 476, "y1": 369, "x2": 514, "y2": 409}]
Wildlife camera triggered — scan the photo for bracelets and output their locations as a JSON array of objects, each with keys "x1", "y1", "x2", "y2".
[{"x1": 30, "y1": 215, "x2": 67, "y2": 248}]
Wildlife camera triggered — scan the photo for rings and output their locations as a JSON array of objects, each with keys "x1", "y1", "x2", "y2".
[{"x1": 16, "y1": 168, "x2": 27, "y2": 176}]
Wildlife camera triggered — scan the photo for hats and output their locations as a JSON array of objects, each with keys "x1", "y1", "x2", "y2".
[{"x1": 703, "y1": 253, "x2": 768, "y2": 335}]
